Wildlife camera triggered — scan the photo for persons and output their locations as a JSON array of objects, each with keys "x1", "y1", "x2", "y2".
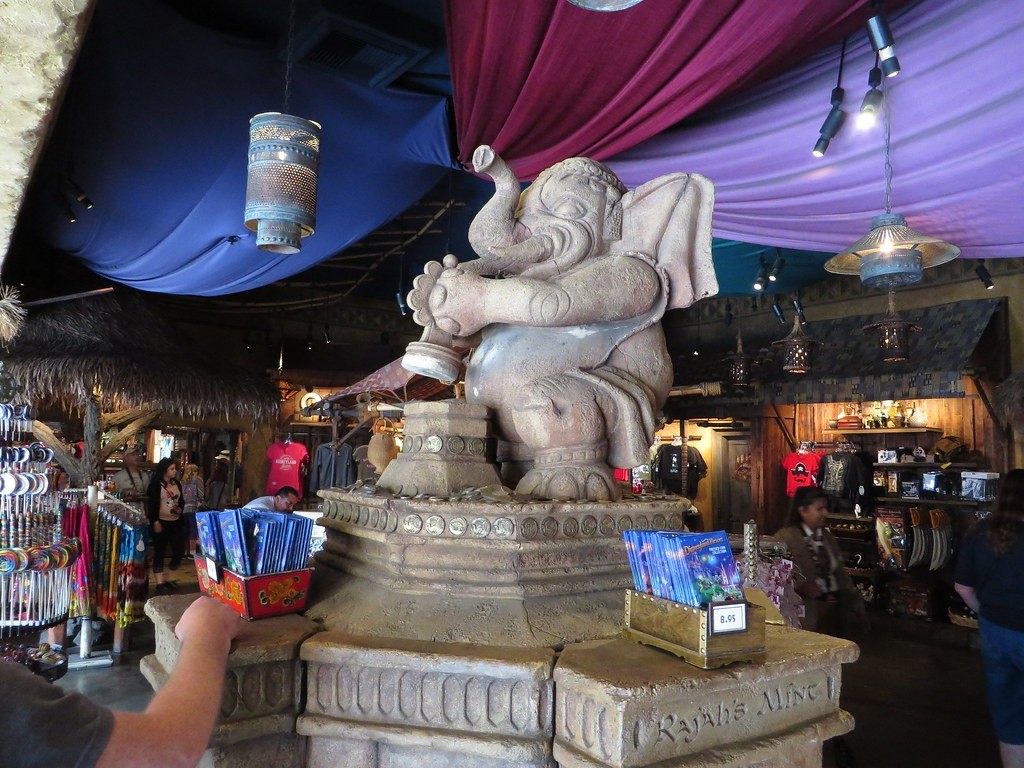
[
  {"x1": 146, "y1": 458, "x2": 185, "y2": 594},
  {"x1": 205, "y1": 466, "x2": 228, "y2": 511},
  {"x1": 955, "y1": 469, "x2": 1024, "y2": 768},
  {"x1": 242, "y1": 485, "x2": 299, "y2": 512},
  {"x1": 0, "y1": 596, "x2": 244, "y2": 768},
  {"x1": 773, "y1": 485, "x2": 847, "y2": 636},
  {"x1": 180, "y1": 464, "x2": 204, "y2": 560},
  {"x1": 112, "y1": 448, "x2": 149, "y2": 517}
]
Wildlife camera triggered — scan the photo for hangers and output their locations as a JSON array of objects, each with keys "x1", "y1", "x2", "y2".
[
  {"x1": 62, "y1": 483, "x2": 150, "y2": 530},
  {"x1": 833, "y1": 439, "x2": 857, "y2": 455},
  {"x1": 795, "y1": 441, "x2": 817, "y2": 454},
  {"x1": 283, "y1": 431, "x2": 292, "y2": 445},
  {"x1": 671, "y1": 437, "x2": 683, "y2": 446},
  {"x1": 329, "y1": 430, "x2": 340, "y2": 442},
  {"x1": 653, "y1": 435, "x2": 662, "y2": 446}
]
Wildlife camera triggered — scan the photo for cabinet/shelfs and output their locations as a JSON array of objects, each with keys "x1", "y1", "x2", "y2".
[{"x1": 822, "y1": 426, "x2": 992, "y2": 506}]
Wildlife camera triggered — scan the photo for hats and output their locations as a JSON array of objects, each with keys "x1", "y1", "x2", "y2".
[{"x1": 123, "y1": 448, "x2": 139, "y2": 458}]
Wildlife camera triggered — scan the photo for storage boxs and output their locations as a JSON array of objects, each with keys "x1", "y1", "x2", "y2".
[
  {"x1": 901, "y1": 481, "x2": 922, "y2": 499},
  {"x1": 623, "y1": 587, "x2": 767, "y2": 671},
  {"x1": 960, "y1": 471, "x2": 999, "y2": 502},
  {"x1": 189, "y1": 551, "x2": 315, "y2": 622},
  {"x1": 885, "y1": 579, "x2": 939, "y2": 622}
]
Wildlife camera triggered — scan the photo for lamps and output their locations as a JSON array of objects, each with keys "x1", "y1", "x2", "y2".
[
  {"x1": 724, "y1": 298, "x2": 733, "y2": 328},
  {"x1": 866, "y1": 15, "x2": 902, "y2": 79},
  {"x1": 820, "y1": 85, "x2": 963, "y2": 289},
  {"x1": 792, "y1": 288, "x2": 807, "y2": 326},
  {"x1": 271, "y1": 312, "x2": 301, "y2": 402},
  {"x1": 51, "y1": 189, "x2": 76, "y2": 223},
  {"x1": 976, "y1": 258, "x2": 995, "y2": 290},
  {"x1": 244, "y1": 0, "x2": 321, "y2": 257},
  {"x1": 754, "y1": 246, "x2": 786, "y2": 290},
  {"x1": 812, "y1": 35, "x2": 849, "y2": 157},
  {"x1": 305, "y1": 385, "x2": 314, "y2": 393},
  {"x1": 771, "y1": 292, "x2": 786, "y2": 325},
  {"x1": 720, "y1": 325, "x2": 758, "y2": 386},
  {"x1": 243, "y1": 333, "x2": 250, "y2": 349},
  {"x1": 65, "y1": 176, "x2": 93, "y2": 210},
  {"x1": 859, "y1": 291, "x2": 923, "y2": 362},
  {"x1": 324, "y1": 292, "x2": 332, "y2": 344},
  {"x1": 770, "y1": 310, "x2": 825, "y2": 374},
  {"x1": 856, "y1": 50, "x2": 884, "y2": 130},
  {"x1": 307, "y1": 294, "x2": 312, "y2": 350}
]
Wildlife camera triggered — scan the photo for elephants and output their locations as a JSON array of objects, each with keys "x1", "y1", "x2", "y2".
[{"x1": 407, "y1": 144, "x2": 721, "y2": 504}]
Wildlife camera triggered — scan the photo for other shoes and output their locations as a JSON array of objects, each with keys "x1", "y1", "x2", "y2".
[
  {"x1": 188, "y1": 554, "x2": 194, "y2": 560},
  {"x1": 167, "y1": 579, "x2": 177, "y2": 591},
  {"x1": 156, "y1": 583, "x2": 168, "y2": 596}
]
